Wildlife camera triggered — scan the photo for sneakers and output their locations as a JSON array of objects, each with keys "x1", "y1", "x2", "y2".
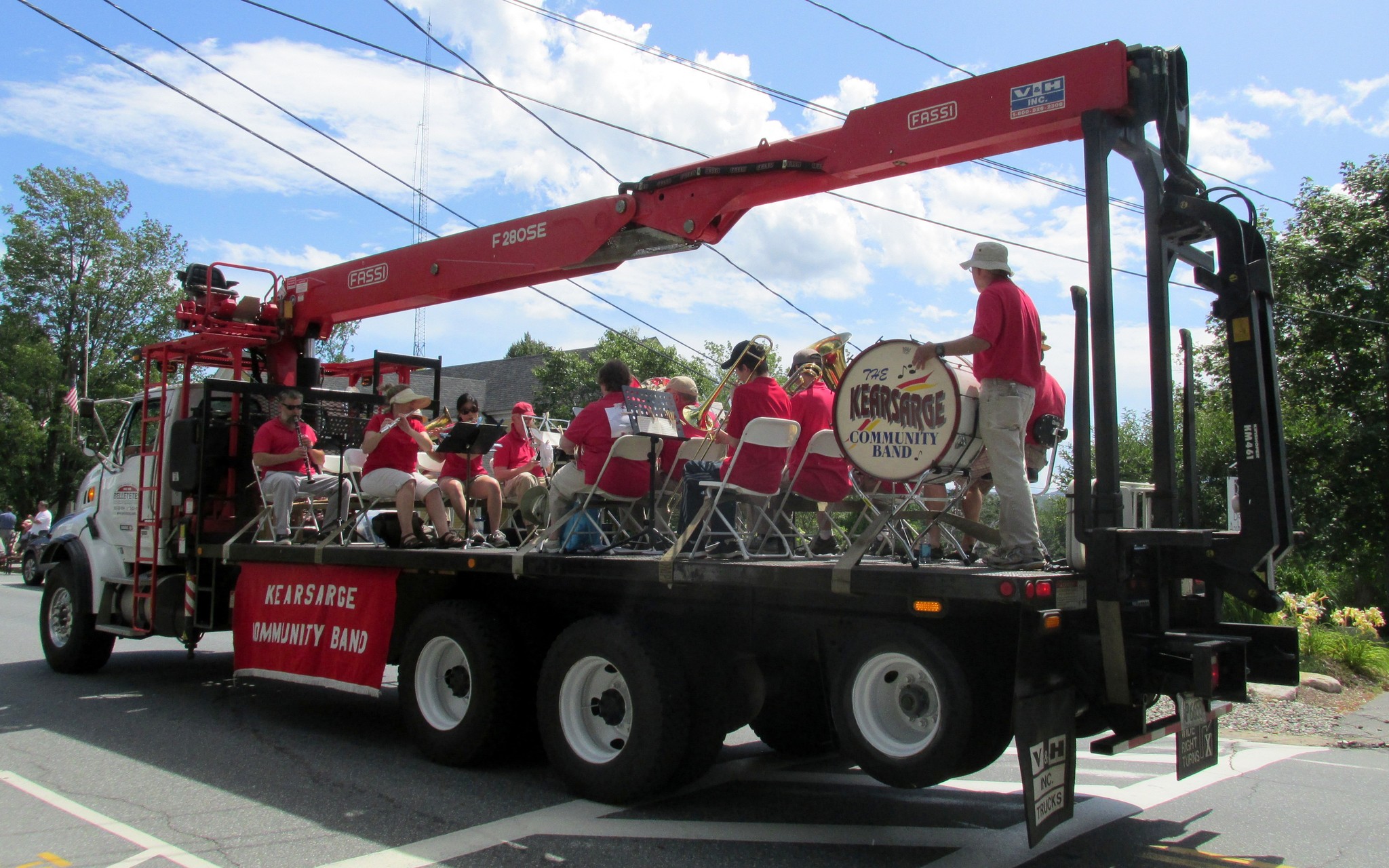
[
  {"x1": 540, "y1": 537, "x2": 561, "y2": 553},
  {"x1": 662, "y1": 540, "x2": 707, "y2": 559},
  {"x1": 468, "y1": 529, "x2": 485, "y2": 546},
  {"x1": 488, "y1": 530, "x2": 510, "y2": 548},
  {"x1": 795, "y1": 535, "x2": 840, "y2": 554},
  {"x1": 909, "y1": 546, "x2": 944, "y2": 562},
  {"x1": 979, "y1": 550, "x2": 1047, "y2": 570},
  {"x1": 708, "y1": 542, "x2": 743, "y2": 559},
  {"x1": 944, "y1": 550, "x2": 971, "y2": 560},
  {"x1": 748, "y1": 536, "x2": 779, "y2": 555},
  {"x1": 276, "y1": 534, "x2": 292, "y2": 545}
]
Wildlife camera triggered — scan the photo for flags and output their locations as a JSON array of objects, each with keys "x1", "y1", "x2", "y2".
[
  {"x1": 63, "y1": 384, "x2": 80, "y2": 416},
  {"x1": 38, "y1": 415, "x2": 53, "y2": 430}
]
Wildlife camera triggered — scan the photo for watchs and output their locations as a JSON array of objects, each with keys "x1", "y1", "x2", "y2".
[{"x1": 935, "y1": 343, "x2": 945, "y2": 357}]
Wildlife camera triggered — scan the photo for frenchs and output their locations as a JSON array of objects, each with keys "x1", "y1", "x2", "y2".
[{"x1": 780, "y1": 332, "x2": 852, "y2": 399}]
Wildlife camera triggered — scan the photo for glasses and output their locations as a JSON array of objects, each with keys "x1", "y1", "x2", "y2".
[
  {"x1": 280, "y1": 401, "x2": 305, "y2": 411},
  {"x1": 458, "y1": 406, "x2": 478, "y2": 415}
]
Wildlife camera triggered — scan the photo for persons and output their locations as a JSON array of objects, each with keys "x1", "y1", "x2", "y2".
[
  {"x1": 360, "y1": 381, "x2": 468, "y2": 548},
  {"x1": 251, "y1": 387, "x2": 352, "y2": 544},
  {"x1": 911, "y1": 329, "x2": 1066, "y2": 562},
  {"x1": 0, "y1": 501, "x2": 52, "y2": 563},
  {"x1": 493, "y1": 402, "x2": 555, "y2": 545},
  {"x1": 422, "y1": 393, "x2": 510, "y2": 548},
  {"x1": 911, "y1": 242, "x2": 1049, "y2": 568},
  {"x1": 619, "y1": 340, "x2": 921, "y2": 558},
  {"x1": 538, "y1": 357, "x2": 660, "y2": 552}
]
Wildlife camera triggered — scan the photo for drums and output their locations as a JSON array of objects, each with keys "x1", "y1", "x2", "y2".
[{"x1": 828, "y1": 334, "x2": 985, "y2": 486}]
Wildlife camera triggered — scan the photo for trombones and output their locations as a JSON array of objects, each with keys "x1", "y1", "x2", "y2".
[{"x1": 665, "y1": 334, "x2": 773, "y2": 515}]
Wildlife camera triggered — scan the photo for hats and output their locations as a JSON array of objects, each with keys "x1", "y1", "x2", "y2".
[
  {"x1": 786, "y1": 348, "x2": 822, "y2": 377},
  {"x1": 1040, "y1": 331, "x2": 1052, "y2": 351},
  {"x1": 959, "y1": 242, "x2": 1015, "y2": 277},
  {"x1": 511, "y1": 401, "x2": 537, "y2": 417},
  {"x1": 721, "y1": 339, "x2": 766, "y2": 369},
  {"x1": 656, "y1": 375, "x2": 699, "y2": 396},
  {"x1": 389, "y1": 388, "x2": 432, "y2": 410}
]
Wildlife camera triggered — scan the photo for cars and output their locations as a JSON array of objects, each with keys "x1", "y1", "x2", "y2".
[{"x1": 21, "y1": 510, "x2": 77, "y2": 585}]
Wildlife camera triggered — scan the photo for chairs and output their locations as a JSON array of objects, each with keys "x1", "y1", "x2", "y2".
[
  {"x1": 538, "y1": 434, "x2": 664, "y2": 556},
  {"x1": 0, "y1": 532, "x2": 18, "y2": 573},
  {"x1": 416, "y1": 452, "x2": 487, "y2": 550},
  {"x1": 345, "y1": 448, "x2": 396, "y2": 549},
  {"x1": 482, "y1": 458, "x2": 525, "y2": 547},
  {"x1": 688, "y1": 418, "x2": 801, "y2": 561},
  {"x1": 250, "y1": 458, "x2": 330, "y2": 545},
  {"x1": 5, "y1": 532, "x2": 32, "y2": 576},
  {"x1": 746, "y1": 429, "x2": 854, "y2": 562},
  {"x1": 610, "y1": 437, "x2": 729, "y2": 552},
  {"x1": 318, "y1": 455, "x2": 371, "y2": 546},
  {"x1": 968, "y1": 413, "x2": 1069, "y2": 563},
  {"x1": 837, "y1": 481, "x2": 922, "y2": 556}
]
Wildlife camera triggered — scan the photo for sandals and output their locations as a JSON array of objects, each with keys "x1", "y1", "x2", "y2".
[
  {"x1": 435, "y1": 532, "x2": 467, "y2": 549},
  {"x1": 399, "y1": 533, "x2": 423, "y2": 549}
]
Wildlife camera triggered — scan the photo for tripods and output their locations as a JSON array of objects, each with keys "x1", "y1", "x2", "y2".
[
  {"x1": 587, "y1": 435, "x2": 676, "y2": 558},
  {"x1": 437, "y1": 453, "x2": 496, "y2": 550},
  {"x1": 299, "y1": 433, "x2": 370, "y2": 547}
]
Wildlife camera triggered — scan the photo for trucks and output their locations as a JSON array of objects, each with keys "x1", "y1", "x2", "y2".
[{"x1": 32, "y1": 39, "x2": 1306, "y2": 852}]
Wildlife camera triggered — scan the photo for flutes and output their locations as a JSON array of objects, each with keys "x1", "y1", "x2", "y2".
[
  {"x1": 428, "y1": 417, "x2": 482, "y2": 434},
  {"x1": 379, "y1": 406, "x2": 419, "y2": 434},
  {"x1": 294, "y1": 416, "x2": 317, "y2": 485},
  {"x1": 525, "y1": 426, "x2": 551, "y2": 490}
]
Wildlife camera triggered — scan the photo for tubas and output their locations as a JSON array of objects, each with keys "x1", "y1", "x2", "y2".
[{"x1": 422, "y1": 405, "x2": 452, "y2": 441}]
